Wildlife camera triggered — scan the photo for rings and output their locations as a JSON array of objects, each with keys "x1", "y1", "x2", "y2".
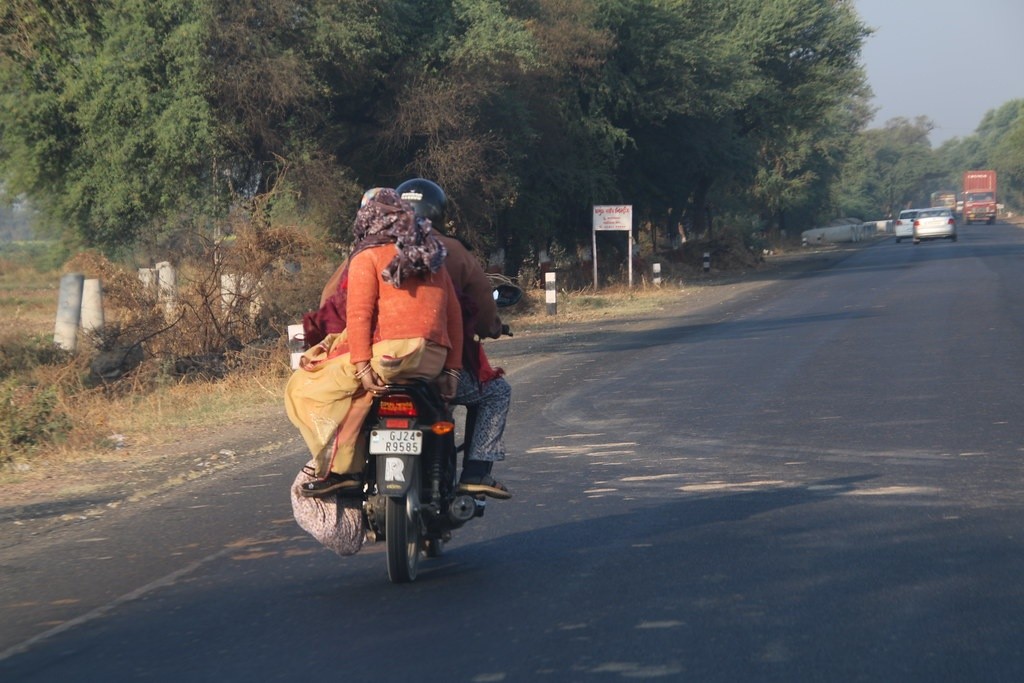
[{"x1": 374, "y1": 390, "x2": 376, "y2": 394}]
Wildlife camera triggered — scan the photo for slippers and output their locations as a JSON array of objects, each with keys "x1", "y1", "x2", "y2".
[{"x1": 458, "y1": 473, "x2": 511, "y2": 499}]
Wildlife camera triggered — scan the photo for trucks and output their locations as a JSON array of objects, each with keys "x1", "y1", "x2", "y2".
[
  {"x1": 961, "y1": 170, "x2": 997, "y2": 226},
  {"x1": 930, "y1": 190, "x2": 957, "y2": 217}
]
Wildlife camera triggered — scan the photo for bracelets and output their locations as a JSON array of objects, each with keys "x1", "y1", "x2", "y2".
[
  {"x1": 442, "y1": 368, "x2": 461, "y2": 380},
  {"x1": 355, "y1": 363, "x2": 371, "y2": 379}
]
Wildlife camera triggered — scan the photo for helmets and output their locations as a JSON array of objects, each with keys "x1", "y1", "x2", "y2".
[{"x1": 395, "y1": 178, "x2": 448, "y2": 233}]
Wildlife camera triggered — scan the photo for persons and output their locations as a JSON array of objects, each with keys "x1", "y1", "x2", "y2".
[
  {"x1": 320, "y1": 177, "x2": 512, "y2": 499},
  {"x1": 283, "y1": 186, "x2": 464, "y2": 496}
]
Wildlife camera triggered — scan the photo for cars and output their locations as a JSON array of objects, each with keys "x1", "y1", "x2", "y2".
[
  {"x1": 911, "y1": 207, "x2": 959, "y2": 245},
  {"x1": 955, "y1": 201, "x2": 963, "y2": 214},
  {"x1": 895, "y1": 209, "x2": 920, "y2": 242}
]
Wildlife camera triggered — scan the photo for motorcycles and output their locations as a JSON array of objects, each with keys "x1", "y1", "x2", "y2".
[{"x1": 363, "y1": 284, "x2": 524, "y2": 581}]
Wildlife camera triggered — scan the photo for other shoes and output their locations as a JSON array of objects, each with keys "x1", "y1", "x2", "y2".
[{"x1": 300, "y1": 472, "x2": 363, "y2": 496}]
